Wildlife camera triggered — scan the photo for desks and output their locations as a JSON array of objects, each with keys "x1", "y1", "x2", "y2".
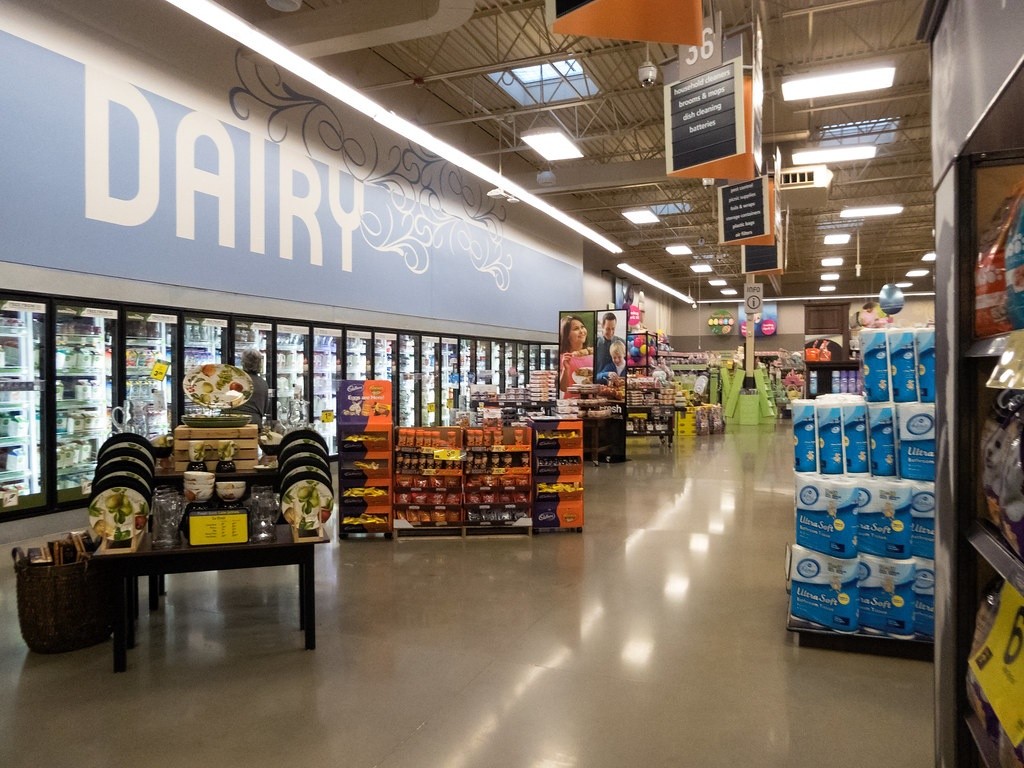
[
  {"x1": 147, "y1": 456, "x2": 278, "y2": 610},
  {"x1": 94, "y1": 524, "x2": 315, "y2": 674}
]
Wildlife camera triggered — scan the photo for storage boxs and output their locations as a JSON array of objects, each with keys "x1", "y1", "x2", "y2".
[
  {"x1": 174, "y1": 438, "x2": 259, "y2": 450},
  {"x1": 174, "y1": 460, "x2": 259, "y2": 471},
  {"x1": 398, "y1": 428, "x2": 456, "y2": 448},
  {"x1": 514, "y1": 429, "x2": 525, "y2": 446},
  {"x1": 467, "y1": 429, "x2": 504, "y2": 446},
  {"x1": 174, "y1": 448, "x2": 258, "y2": 461},
  {"x1": 529, "y1": 370, "x2": 558, "y2": 402},
  {"x1": 174, "y1": 423, "x2": 259, "y2": 439},
  {"x1": 5, "y1": 447, "x2": 28, "y2": 472},
  {"x1": 627, "y1": 381, "x2": 713, "y2": 436}
]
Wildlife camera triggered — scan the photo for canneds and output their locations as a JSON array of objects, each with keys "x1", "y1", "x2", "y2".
[
  {"x1": 465, "y1": 452, "x2": 529, "y2": 470},
  {"x1": 395, "y1": 452, "x2": 460, "y2": 469}
]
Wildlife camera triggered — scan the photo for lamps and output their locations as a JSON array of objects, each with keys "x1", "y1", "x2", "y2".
[
  {"x1": 818, "y1": 284, "x2": 837, "y2": 292},
  {"x1": 638, "y1": 42, "x2": 658, "y2": 90},
  {"x1": 374, "y1": 110, "x2": 624, "y2": 256},
  {"x1": 689, "y1": 260, "x2": 713, "y2": 273},
  {"x1": 780, "y1": 59, "x2": 896, "y2": 102},
  {"x1": 619, "y1": 207, "x2": 660, "y2": 224},
  {"x1": 487, "y1": 125, "x2": 520, "y2": 204},
  {"x1": 823, "y1": 231, "x2": 851, "y2": 245},
  {"x1": 820, "y1": 271, "x2": 840, "y2": 281},
  {"x1": 664, "y1": 243, "x2": 693, "y2": 256},
  {"x1": 821, "y1": 256, "x2": 844, "y2": 267},
  {"x1": 920, "y1": 251, "x2": 936, "y2": 261},
  {"x1": 537, "y1": 171, "x2": 558, "y2": 188},
  {"x1": 791, "y1": 142, "x2": 878, "y2": 167},
  {"x1": 166, "y1": 0, "x2": 387, "y2": 120},
  {"x1": 617, "y1": 262, "x2": 696, "y2": 304},
  {"x1": 895, "y1": 280, "x2": 914, "y2": 289},
  {"x1": 720, "y1": 287, "x2": 738, "y2": 295},
  {"x1": 520, "y1": 126, "x2": 584, "y2": 161},
  {"x1": 905, "y1": 267, "x2": 930, "y2": 277},
  {"x1": 840, "y1": 204, "x2": 904, "y2": 218},
  {"x1": 707, "y1": 277, "x2": 727, "y2": 286}
]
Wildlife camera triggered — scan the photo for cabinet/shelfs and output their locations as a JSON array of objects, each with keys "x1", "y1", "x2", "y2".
[
  {"x1": 470, "y1": 399, "x2": 557, "y2": 426},
  {"x1": 392, "y1": 425, "x2": 465, "y2": 540},
  {"x1": 532, "y1": 420, "x2": 585, "y2": 536},
  {"x1": 626, "y1": 406, "x2": 676, "y2": 449},
  {"x1": 803, "y1": 302, "x2": 863, "y2": 399},
  {"x1": 336, "y1": 379, "x2": 393, "y2": 541},
  {"x1": 462, "y1": 426, "x2": 534, "y2": 539},
  {"x1": 577, "y1": 392, "x2": 615, "y2": 467},
  {"x1": 627, "y1": 331, "x2": 658, "y2": 377},
  {"x1": 915, "y1": 0, "x2": 1024, "y2": 768}
]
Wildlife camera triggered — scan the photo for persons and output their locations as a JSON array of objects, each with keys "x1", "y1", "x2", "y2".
[
  {"x1": 560, "y1": 315, "x2": 593, "y2": 399},
  {"x1": 597, "y1": 340, "x2": 626, "y2": 385},
  {"x1": 219, "y1": 349, "x2": 269, "y2": 433},
  {"x1": 597, "y1": 313, "x2": 626, "y2": 372}
]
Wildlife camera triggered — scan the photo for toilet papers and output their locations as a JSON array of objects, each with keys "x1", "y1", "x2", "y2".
[{"x1": 786, "y1": 329, "x2": 938, "y2": 640}]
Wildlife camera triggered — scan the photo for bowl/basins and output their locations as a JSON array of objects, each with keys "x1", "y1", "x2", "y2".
[
  {"x1": 215, "y1": 482, "x2": 246, "y2": 502},
  {"x1": 152, "y1": 445, "x2": 173, "y2": 457},
  {"x1": 183, "y1": 471, "x2": 215, "y2": 503},
  {"x1": 260, "y1": 445, "x2": 279, "y2": 456}
]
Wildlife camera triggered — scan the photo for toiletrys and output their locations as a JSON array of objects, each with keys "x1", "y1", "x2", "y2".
[
  {"x1": 809, "y1": 370, "x2": 818, "y2": 394},
  {"x1": 832, "y1": 371, "x2": 840, "y2": 394},
  {"x1": 857, "y1": 371, "x2": 863, "y2": 394},
  {"x1": 848, "y1": 371, "x2": 856, "y2": 394},
  {"x1": 840, "y1": 371, "x2": 847, "y2": 394}
]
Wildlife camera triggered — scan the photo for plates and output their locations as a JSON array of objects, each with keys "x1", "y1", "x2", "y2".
[
  {"x1": 277, "y1": 430, "x2": 333, "y2": 530},
  {"x1": 88, "y1": 432, "x2": 156, "y2": 541},
  {"x1": 180, "y1": 414, "x2": 251, "y2": 427},
  {"x1": 571, "y1": 366, "x2": 593, "y2": 384},
  {"x1": 182, "y1": 364, "x2": 253, "y2": 408}
]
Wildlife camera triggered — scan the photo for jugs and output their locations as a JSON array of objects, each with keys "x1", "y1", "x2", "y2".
[
  {"x1": 276, "y1": 400, "x2": 310, "y2": 430},
  {"x1": 112, "y1": 398, "x2": 147, "y2": 440}
]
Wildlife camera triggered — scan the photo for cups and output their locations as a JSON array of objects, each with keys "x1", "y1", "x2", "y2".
[
  {"x1": 188, "y1": 440, "x2": 214, "y2": 463},
  {"x1": 250, "y1": 486, "x2": 279, "y2": 541},
  {"x1": 216, "y1": 440, "x2": 240, "y2": 461},
  {"x1": 151, "y1": 487, "x2": 190, "y2": 550}
]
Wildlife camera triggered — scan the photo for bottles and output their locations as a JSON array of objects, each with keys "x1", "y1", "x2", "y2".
[
  {"x1": 466, "y1": 454, "x2": 488, "y2": 469},
  {"x1": 398, "y1": 428, "x2": 456, "y2": 447},
  {"x1": 56, "y1": 379, "x2": 99, "y2": 468},
  {"x1": 491, "y1": 454, "x2": 500, "y2": 467},
  {"x1": 396, "y1": 452, "x2": 459, "y2": 469},
  {"x1": 539, "y1": 459, "x2": 578, "y2": 465},
  {"x1": 646, "y1": 420, "x2": 665, "y2": 427},
  {"x1": 514, "y1": 429, "x2": 524, "y2": 445},
  {"x1": 634, "y1": 417, "x2": 645, "y2": 431},
  {"x1": 809, "y1": 370, "x2": 861, "y2": 393},
  {"x1": 522, "y1": 453, "x2": 529, "y2": 467},
  {"x1": 501, "y1": 454, "x2": 512, "y2": 468},
  {"x1": 467, "y1": 429, "x2": 503, "y2": 446}
]
